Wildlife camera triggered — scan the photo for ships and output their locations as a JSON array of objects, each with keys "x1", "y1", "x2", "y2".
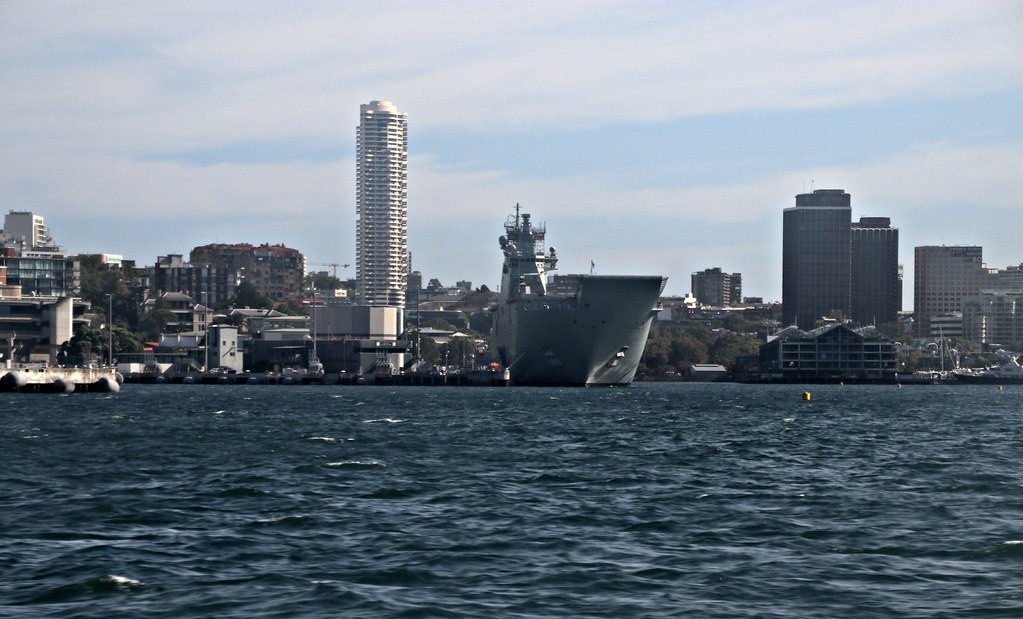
[{"x1": 489, "y1": 203, "x2": 670, "y2": 385}]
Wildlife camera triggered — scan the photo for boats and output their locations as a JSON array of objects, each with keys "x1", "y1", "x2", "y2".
[
  {"x1": 951, "y1": 350, "x2": 1023, "y2": 381},
  {"x1": 462, "y1": 366, "x2": 511, "y2": 385}
]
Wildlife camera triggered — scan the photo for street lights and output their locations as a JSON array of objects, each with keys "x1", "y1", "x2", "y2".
[
  {"x1": 200, "y1": 290, "x2": 208, "y2": 373},
  {"x1": 105, "y1": 293, "x2": 112, "y2": 368}
]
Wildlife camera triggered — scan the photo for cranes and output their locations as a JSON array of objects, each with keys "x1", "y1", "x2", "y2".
[{"x1": 318, "y1": 262, "x2": 351, "y2": 277}]
